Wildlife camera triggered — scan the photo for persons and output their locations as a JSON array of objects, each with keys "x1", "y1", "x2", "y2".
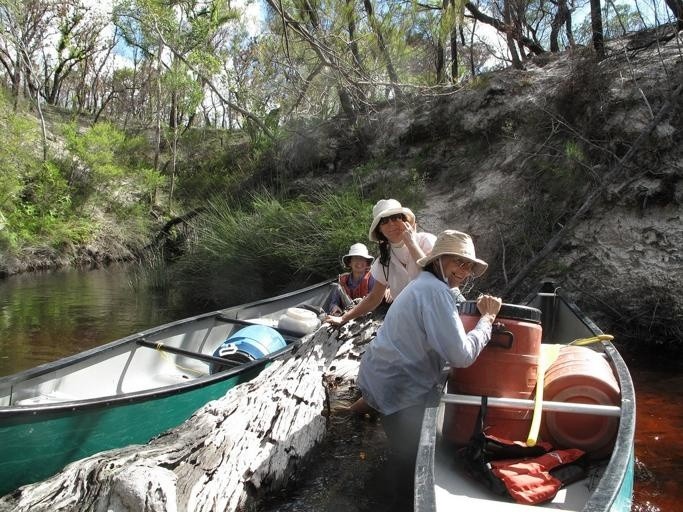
[
  {"x1": 323, "y1": 242, "x2": 393, "y2": 318},
  {"x1": 356, "y1": 229, "x2": 501, "y2": 450},
  {"x1": 323, "y1": 199, "x2": 437, "y2": 328}
]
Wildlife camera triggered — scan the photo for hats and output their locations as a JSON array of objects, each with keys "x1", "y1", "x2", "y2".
[
  {"x1": 417, "y1": 229, "x2": 488, "y2": 278},
  {"x1": 342, "y1": 242, "x2": 374, "y2": 268},
  {"x1": 369, "y1": 199, "x2": 416, "y2": 242}
]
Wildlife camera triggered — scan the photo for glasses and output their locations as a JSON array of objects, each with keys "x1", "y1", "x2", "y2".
[
  {"x1": 379, "y1": 214, "x2": 405, "y2": 225},
  {"x1": 449, "y1": 255, "x2": 473, "y2": 270}
]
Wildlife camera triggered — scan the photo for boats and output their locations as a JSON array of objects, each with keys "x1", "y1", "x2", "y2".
[
  {"x1": 413, "y1": 278, "x2": 637, "y2": 512},
  {"x1": 0, "y1": 269, "x2": 352, "y2": 494}
]
